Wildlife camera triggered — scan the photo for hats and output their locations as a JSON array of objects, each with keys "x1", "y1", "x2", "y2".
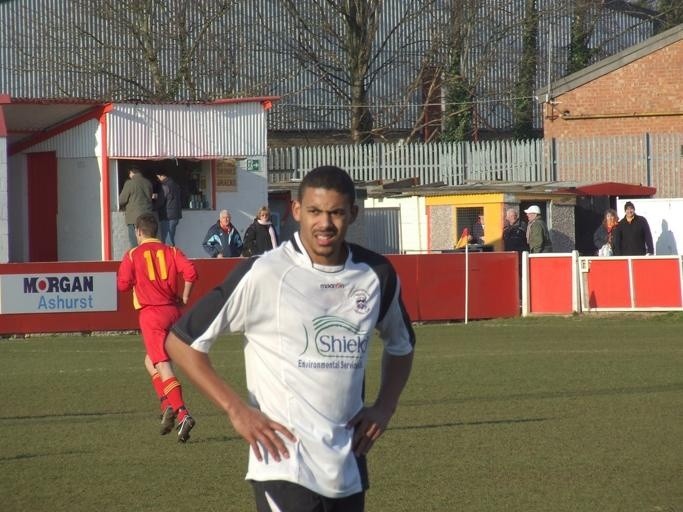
[
  {"x1": 524, "y1": 206, "x2": 541, "y2": 214},
  {"x1": 625, "y1": 201, "x2": 636, "y2": 211}
]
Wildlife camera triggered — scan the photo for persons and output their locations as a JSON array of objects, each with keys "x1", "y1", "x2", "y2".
[
  {"x1": 116, "y1": 165, "x2": 155, "y2": 249},
  {"x1": 242, "y1": 206, "x2": 280, "y2": 258},
  {"x1": 114, "y1": 212, "x2": 197, "y2": 444},
  {"x1": 163, "y1": 166, "x2": 414, "y2": 510},
  {"x1": 203, "y1": 210, "x2": 242, "y2": 256},
  {"x1": 152, "y1": 170, "x2": 186, "y2": 247},
  {"x1": 469, "y1": 200, "x2": 656, "y2": 255}
]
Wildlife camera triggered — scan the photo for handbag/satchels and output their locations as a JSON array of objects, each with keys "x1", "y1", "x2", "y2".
[{"x1": 599, "y1": 242, "x2": 613, "y2": 256}]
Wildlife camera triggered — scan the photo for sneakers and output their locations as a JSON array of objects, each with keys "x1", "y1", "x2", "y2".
[
  {"x1": 177, "y1": 414, "x2": 194, "y2": 442},
  {"x1": 159, "y1": 406, "x2": 174, "y2": 435}
]
